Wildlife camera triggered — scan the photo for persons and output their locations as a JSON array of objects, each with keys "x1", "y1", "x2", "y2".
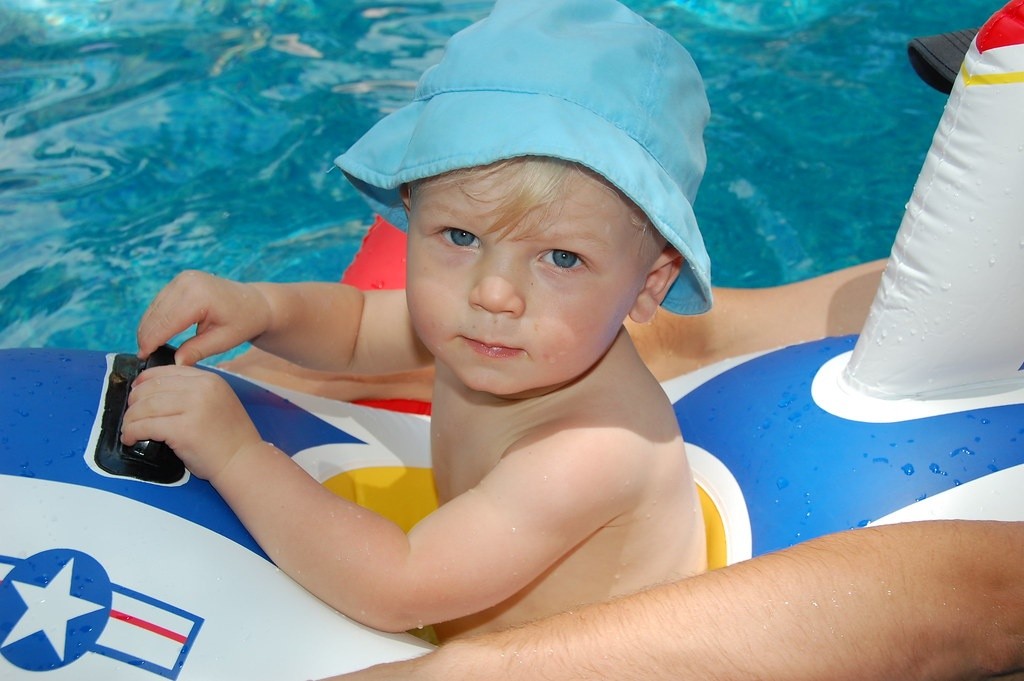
[
  {"x1": 214, "y1": 257, "x2": 1024, "y2": 681},
  {"x1": 120, "y1": 0, "x2": 712, "y2": 646}
]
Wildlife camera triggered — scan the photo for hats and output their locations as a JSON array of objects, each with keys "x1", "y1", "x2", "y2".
[
  {"x1": 908, "y1": 27, "x2": 980, "y2": 96},
  {"x1": 334, "y1": 0, "x2": 713, "y2": 315}
]
